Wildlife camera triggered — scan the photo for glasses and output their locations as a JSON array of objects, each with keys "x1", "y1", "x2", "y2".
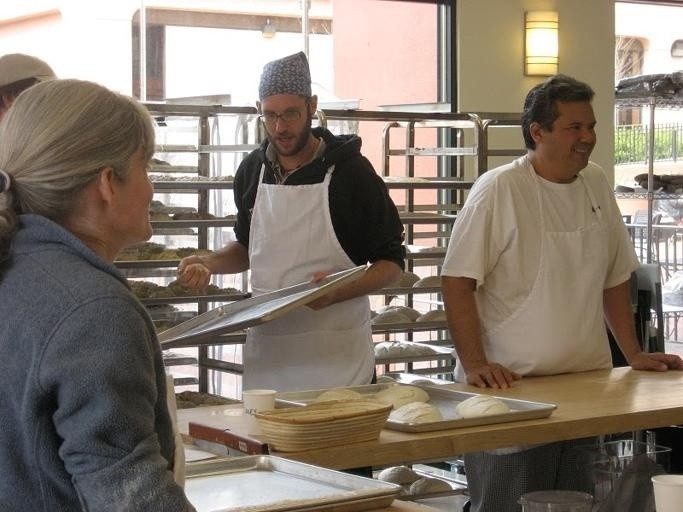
[{"x1": 257, "y1": 102, "x2": 308, "y2": 124}]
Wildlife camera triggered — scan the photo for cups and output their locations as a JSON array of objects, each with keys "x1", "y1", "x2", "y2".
[{"x1": 241, "y1": 388, "x2": 276, "y2": 435}]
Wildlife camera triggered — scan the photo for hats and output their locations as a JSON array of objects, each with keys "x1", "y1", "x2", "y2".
[
  {"x1": 259, "y1": 51, "x2": 312, "y2": 100},
  {"x1": 0, "y1": 54, "x2": 58, "y2": 82}
]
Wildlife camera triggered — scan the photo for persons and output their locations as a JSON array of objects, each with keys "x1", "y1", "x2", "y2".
[
  {"x1": 0, "y1": 53, "x2": 59, "y2": 139},
  {"x1": 440, "y1": 75, "x2": 683, "y2": 511},
  {"x1": 0, "y1": 79, "x2": 196, "y2": 511},
  {"x1": 179, "y1": 53, "x2": 407, "y2": 480}
]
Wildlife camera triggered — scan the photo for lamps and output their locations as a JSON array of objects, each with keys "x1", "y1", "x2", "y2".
[{"x1": 519, "y1": 7, "x2": 563, "y2": 80}]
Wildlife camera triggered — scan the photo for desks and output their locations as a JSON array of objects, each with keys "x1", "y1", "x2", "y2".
[{"x1": 173, "y1": 362, "x2": 681, "y2": 512}]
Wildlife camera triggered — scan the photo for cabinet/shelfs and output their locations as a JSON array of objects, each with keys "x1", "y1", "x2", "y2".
[
  {"x1": 94, "y1": 98, "x2": 531, "y2": 410},
  {"x1": 609, "y1": 88, "x2": 681, "y2": 346}
]
